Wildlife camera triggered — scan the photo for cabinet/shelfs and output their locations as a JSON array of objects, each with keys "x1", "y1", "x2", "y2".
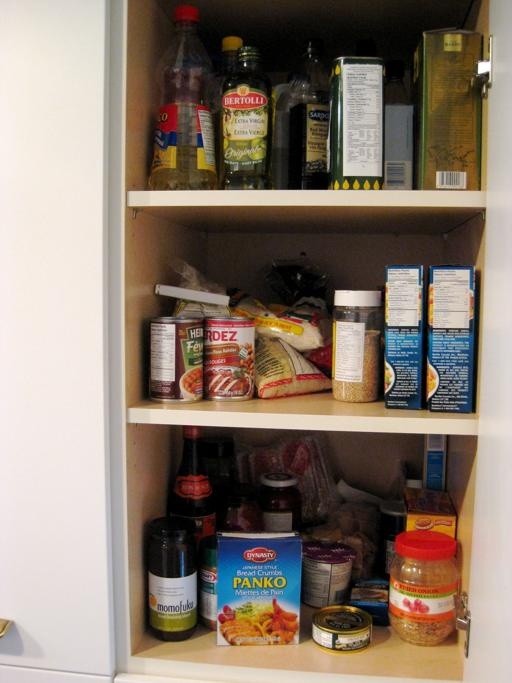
[{"x1": 121, "y1": 187, "x2": 485, "y2": 683}]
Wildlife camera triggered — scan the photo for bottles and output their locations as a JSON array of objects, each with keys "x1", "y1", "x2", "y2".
[
  {"x1": 169, "y1": 426, "x2": 217, "y2": 542},
  {"x1": 149, "y1": 4, "x2": 330, "y2": 204}
]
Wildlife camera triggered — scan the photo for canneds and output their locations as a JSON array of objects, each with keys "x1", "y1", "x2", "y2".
[
  {"x1": 311, "y1": 604, "x2": 373, "y2": 652},
  {"x1": 200, "y1": 535, "x2": 217, "y2": 631},
  {"x1": 258, "y1": 471, "x2": 303, "y2": 532},
  {"x1": 146, "y1": 317, "x2": 204, "y2": 404},
  {"x1": 376, "y1": 503, "x2": 406, "y2": 579},
  {"x1": 202, "y1": 317, "x2": 257, "y2": 403},
  {"x1": 146, "y1": 516, "x2": 202, "y2": 642}
]
